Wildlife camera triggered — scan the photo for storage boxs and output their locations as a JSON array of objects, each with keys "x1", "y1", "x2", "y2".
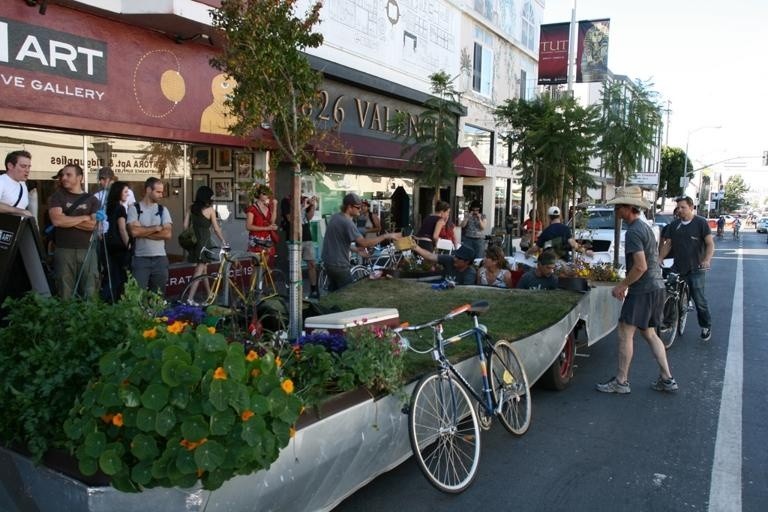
[{"x1": 304, "y1": 308, "x2": 400, "y2": 338}]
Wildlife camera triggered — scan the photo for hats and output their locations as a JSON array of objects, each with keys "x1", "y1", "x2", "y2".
[
  {"x1": 606, "y1": 186, "x2": 651, "y2": 210},
  {"x1": 343, "y1": 194, "x2": 360, "y2": 204},
  {"x1": 454, "y1": 246, "x2": 475, "y2": 260},
  {"x1": 549, "y1": 206, "x2": 560, "y2": 216},
  {"x1": 534, "y1": 253, "x2": 555, "y2": 264}
]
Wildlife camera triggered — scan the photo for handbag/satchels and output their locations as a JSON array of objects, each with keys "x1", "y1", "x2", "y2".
[
  {"x1": 267, "y1": 223, "x2": 281, "y2": 243},
  {"x1": 179, "y1": 231, "x2": 197, "y2": 249}
]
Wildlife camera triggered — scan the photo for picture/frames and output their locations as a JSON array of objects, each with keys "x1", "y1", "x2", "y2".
[{"x1": 191, "y1": 145, "x2": 255, "y2": 220}]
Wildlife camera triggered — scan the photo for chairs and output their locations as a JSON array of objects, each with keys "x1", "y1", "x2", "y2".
[
  {"x1": 509, "y1": 271, "x2": 523, "y2": 288},
  {"x1": 558, "y1": 277, "x2": 588, "y2": 339}
]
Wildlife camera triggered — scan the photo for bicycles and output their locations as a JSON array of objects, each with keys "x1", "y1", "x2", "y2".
[
  {"x1": 180, "y1": 239, "x2": 287, "y2": 307},
  {"x1": 318, "y1": 232, "x2": 410, "y2": 295},
  {"x1": 716, "y1": 224, "x2": 725, "y2": 239},
  {"x1": 733, "y1": 226, "x2": 740, "y2": 239},
  {"x1": 392, "y1": 301, "x2": 531, "y2": 493},
  {"x1": 657, "y1": 263, "x2": 693, "y2": 348}
]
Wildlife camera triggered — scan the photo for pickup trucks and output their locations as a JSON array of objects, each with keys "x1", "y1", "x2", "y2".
[{"x1": 562, "y1": 208, "x2": 660, "y2": 273}]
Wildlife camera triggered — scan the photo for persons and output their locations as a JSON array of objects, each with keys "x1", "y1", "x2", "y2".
[
  {"x1": 280, "y1": 189, "x2": 403, "y2": 299},
  {"x1": 653, "y1": 195, "x2": 713, "y2": 344},
  {"x1": 658, "y1": 206, "x2": 693, "y2": 314},
  {"x1": 246, "y1": 184, "x2": 278, "y2": 291},
  {"x1": 409, "y1": 199, "x2": 486, "y2": 285},
  {"x1": 513, "y1": 234, "x2": 571, "y2": 290},
  {"x1": 714, "y1": 209, "x2": 761, "y2": 241},
  {"x1": 183, "y1": 184, "x2": 229, "y2": 307},
  {"x1": 0, "y1": 150, "x2": 173, "y2": 305},
  {"x1": 476, "y1": 244, "x2": 512, "y2": 289},
  {"x1": 506, "y1": 206, "x2": 543, "y2": 242},
  {"x1": 525, "y1": 206, "x2": 593, "y2": 267},
  {"x1": 596, "y1": 185, "x2": 680, "y2": 393},
  {"x1": 569, "y1": 206, "x2": 574, "y2": 222}
]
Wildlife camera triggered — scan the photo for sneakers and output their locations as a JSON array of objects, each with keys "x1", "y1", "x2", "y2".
[
  {"x1": 660, "y1": 324, "x2": 672, "y2": 332},
  {"x1": 651, "y1": 375, "x2": 678, "y2": 390},
  {"x1": 701, "y1": 328, "x2": 711, "y2": 341},
  {"x1": 596, "y1": 377, "x2": 631, "y2": 393}
]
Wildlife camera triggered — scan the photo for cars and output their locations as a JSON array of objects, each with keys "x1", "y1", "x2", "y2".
[{"x1": 757, "y1": 217, "x2": 768, "y2": 233}]
